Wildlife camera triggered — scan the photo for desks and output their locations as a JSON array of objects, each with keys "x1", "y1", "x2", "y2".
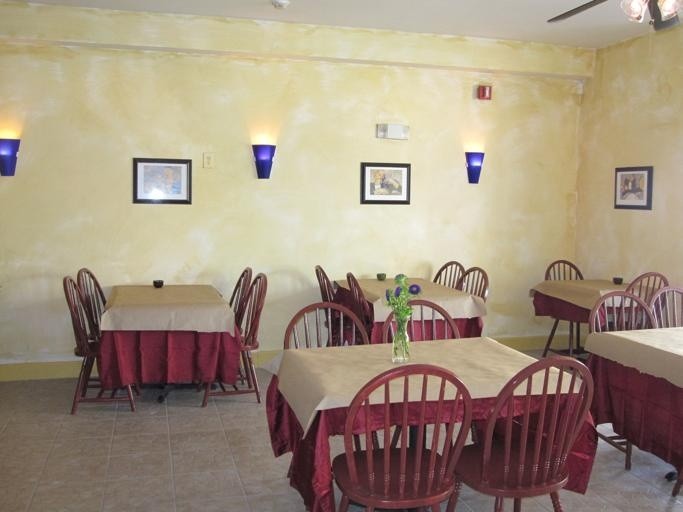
[
  {"x1": 249, "y1": 338, "x2": 598, "y2": 512},
  {"x1": 335, "y1": 279, "x2": 485, "y2": 344},
  {"x1": 584, "y1": 327, "x2": 683, "y2": 498},
  {"x1": 99, "y1": 284, "x2": 241, "y2": 401},
  {"x1": 529, "y1": 279, "x2": 663, "y2": 357}
]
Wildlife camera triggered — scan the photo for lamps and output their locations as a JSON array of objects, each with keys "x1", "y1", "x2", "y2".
[
  {"x1": 0, "y1": 129, "x2": 21, "y2": 176},
  {"x1": 621, "y1": 0, "x2": 679, "y2": 29},
  {"x1": 252, "y1": 145, "x2": 276, "y2": 180},
  {"x1": 465, "y1": 153, "x2": 485, "y2": 184}
]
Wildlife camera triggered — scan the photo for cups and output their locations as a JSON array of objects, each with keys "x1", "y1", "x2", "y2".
[
  {"x1": 613, "y1": 277, "x2": 623, "y2": 284},
  {"x1": 153, "y1": 280, "x2": 164, "y2": 288},
  {"x1": 376, "y1": 274, "x2": 386, "y2": 280}
]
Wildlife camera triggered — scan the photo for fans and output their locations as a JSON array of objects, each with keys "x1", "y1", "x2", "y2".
[{"x1": 547, "y1": 0, "x2": 679, "y2": 31}]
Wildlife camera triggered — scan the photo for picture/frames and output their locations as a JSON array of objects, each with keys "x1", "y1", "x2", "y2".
[
  {"x1": 132, "y1": 158, "x2": 192, "y2": 204},
  {"x1": 614, "y1": 167, "x2": 652, "y2": 210},
  {"x1": 359, "y1": 162, "x2": 410, "y2": 204}
]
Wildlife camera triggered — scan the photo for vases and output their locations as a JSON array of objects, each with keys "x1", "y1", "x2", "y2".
[{"x1": 393, "y1": 316, "x2": 410, "y2": 363}]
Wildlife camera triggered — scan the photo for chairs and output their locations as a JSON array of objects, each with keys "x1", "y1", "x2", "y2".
[
  {"x1": 63, "y1": 268, "x2": 140, "y2": 414},
  {"x1": 588, "y1": 272, "x2": 683, "y2": 333},
  {"x1": 543, "y1": 261, "x2": 587, "y2": 358},
  {"x1": 197, "y1": 268, "x2": 267, "y2": 405},
  {"x1": 432, "y1": 261, "x2": 489, "y2": 299},
  {"x1": 285, "y1": 266, "x2": 373, "y2": 348}
]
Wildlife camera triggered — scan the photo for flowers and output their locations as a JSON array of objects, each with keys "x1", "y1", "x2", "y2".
[{"x1": 386, "y1": 275, "x2": 421, "y2": 319}]
